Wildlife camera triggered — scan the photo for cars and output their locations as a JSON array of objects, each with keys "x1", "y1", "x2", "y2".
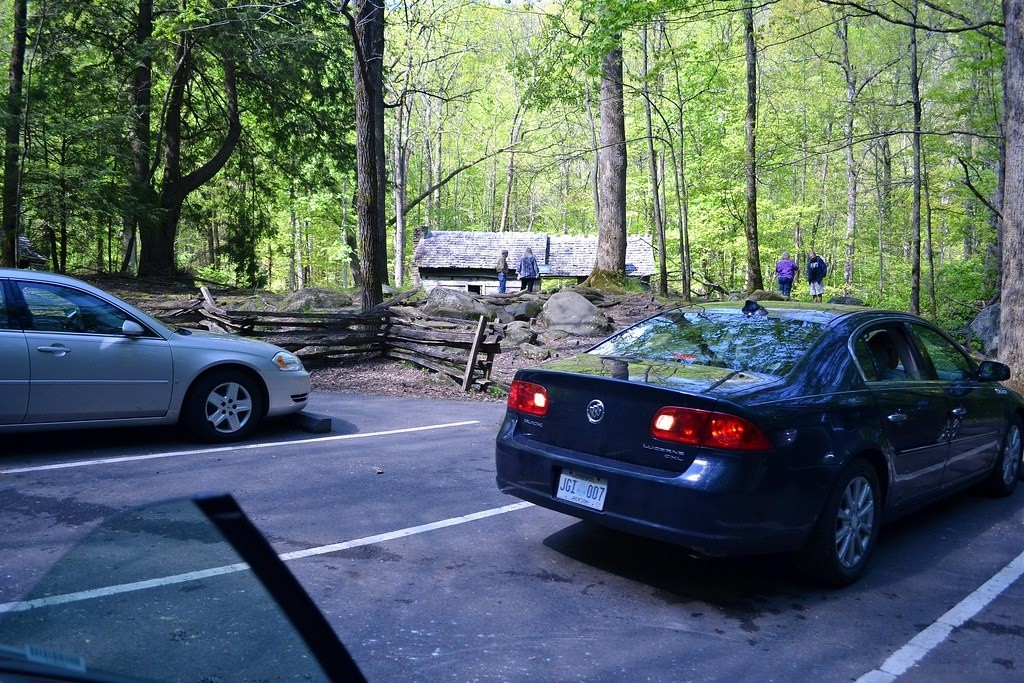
[
  {"x1": 1, "y1": 271, "x2": 313, "y2": 454},
  {"x1": 495, "y1": 303, "x2": 1024, "y2": 587}
]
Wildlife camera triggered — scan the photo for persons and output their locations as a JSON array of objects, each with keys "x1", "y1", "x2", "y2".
[
  {"x1": 807, "y1": 251, "x2": 827, "y2": 303},
  {"x1": 776, "y1": 252, "x2": 799, "y2": 297},
  {"x1": 496, "y1": 250, "x2": 509, "y2": 295},
  {"x1": 515, "y1": 248, "x2": 539, "y2": 292}
]
